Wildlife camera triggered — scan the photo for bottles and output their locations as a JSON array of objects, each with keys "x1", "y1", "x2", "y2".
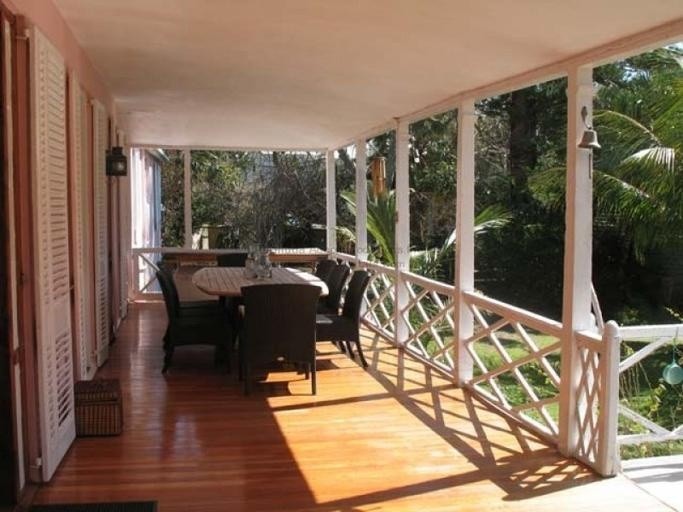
[{"x1": 243, "y1": 243, "x2": 273, "y2": 281}]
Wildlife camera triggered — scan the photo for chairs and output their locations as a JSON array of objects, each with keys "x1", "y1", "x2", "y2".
[{"x1": 150, "y1": 253, "x2": 370, "y2": 400}]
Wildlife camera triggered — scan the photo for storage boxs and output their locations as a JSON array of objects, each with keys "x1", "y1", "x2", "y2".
[{"x1": 73, "y1": 376, "x2": 122, "y2": 436}]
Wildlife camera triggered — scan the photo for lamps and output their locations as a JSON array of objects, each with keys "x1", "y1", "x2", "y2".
[{"x1": 103, "y1": 144, "x2": 128, "y2": 176}]
